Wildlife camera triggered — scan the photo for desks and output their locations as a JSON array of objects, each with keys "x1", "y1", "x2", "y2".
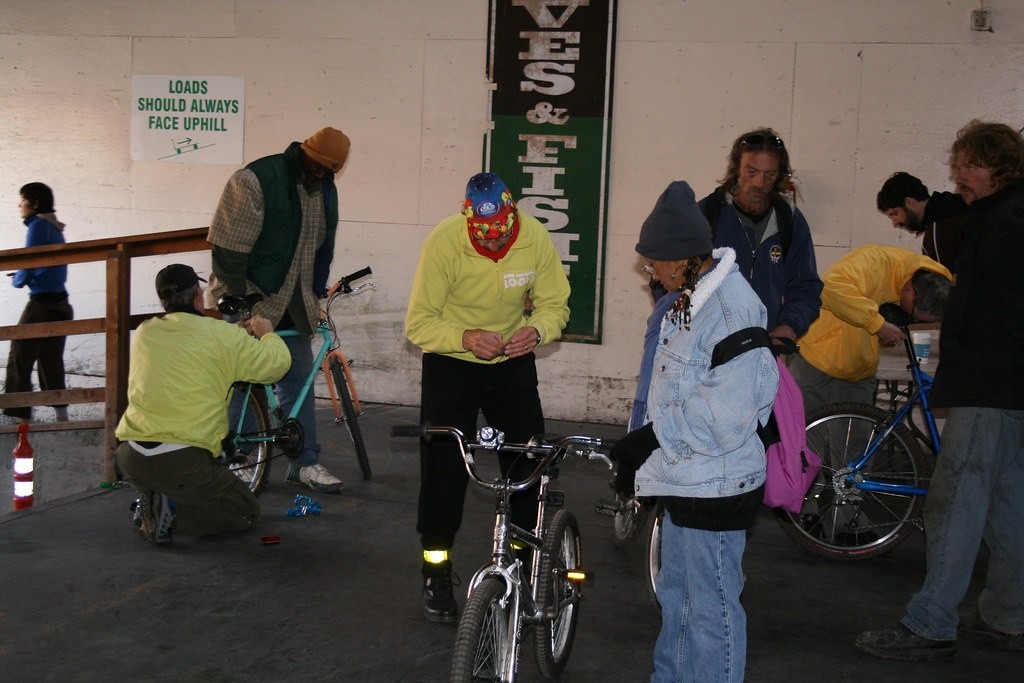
[{"x1": 875, "y1": 330, "x2": 943, "y2": 491}]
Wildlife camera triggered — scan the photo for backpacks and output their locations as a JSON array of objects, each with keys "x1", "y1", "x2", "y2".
[{"x1": 708, "y1": 327, "x2": 822, "y2": 514}]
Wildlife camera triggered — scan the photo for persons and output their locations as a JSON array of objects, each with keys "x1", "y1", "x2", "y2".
[
  {"x1": 3, "y1": 182, "x2": 73, "y2": 424},
  {"x1": 649, "y1": 130, "x2": 824, "y2": 363},
  {"x1": 115, "y1": 264, "x2": 291, "y2": 543},
  {"x1": 855, "y1": 122, "x2": 1024, "y2": 661},
  {"x1": 405, "y1": 171, "x2": 571, "y2": 619},
  {"x1": 877, "y1": 172, "x2": 968, "y2": 258},
  {"x1": 787, "y1": 245, "x2": 955, "y2": 547},
  {"x1": 608, "y1": 181, "x2": 779, "y2": 683},
  {"x1": 207, "y1": 127, "x2": 351, "y2": 492}
]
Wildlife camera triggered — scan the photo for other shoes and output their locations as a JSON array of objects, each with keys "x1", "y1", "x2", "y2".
[
  {"x1": 837, "y1": 531, "x2": 881, "y2": 547},
  {"x1": 802, "y1": 517, "x2": 822, "y2": 539}
]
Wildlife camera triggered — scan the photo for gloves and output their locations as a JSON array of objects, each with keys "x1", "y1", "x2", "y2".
[{"x1": 609, "y1": 421, "x2": 659, "y2": 496}]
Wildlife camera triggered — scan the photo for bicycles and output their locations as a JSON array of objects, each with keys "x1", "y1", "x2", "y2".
[
  {"x1": 389, "y1": 419, "x2": 619, "y2": 683},
  {"x1": 595, "y1": 490, "x2": 662, "y2": 617},
  {"x1": 775, "y1": 302, "x2": 942, "y2": 565},
  {"x1": 217, "y1": 265, "x2": 376, "y2": 499}
]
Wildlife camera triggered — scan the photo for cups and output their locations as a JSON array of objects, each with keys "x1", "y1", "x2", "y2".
[{"x1": 913, "y1": 333, "x2": 931, "y2": 364}]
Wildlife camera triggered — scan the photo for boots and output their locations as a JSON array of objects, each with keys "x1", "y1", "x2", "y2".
[{"x1": 420, "y1": 560, "x2": 462, "y2": 624}]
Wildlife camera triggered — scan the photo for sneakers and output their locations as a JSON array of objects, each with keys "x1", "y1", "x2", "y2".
[
  {"x1": 131, "y1": 493, "x2": 157, "y2": 543},
  {"x1": 151, "y1": 492, "x2": 178, "y2": 547},
  {"x1": 286, "y1": 464, "x2": 342, "y2": 494},
  {"x1": 229, "y1": 458, "x2": 255, "y2": 484},
  {"x1": 958, "y1": 611, "x2": 1024, "y2": 652},
  {"x1": 856, "y1": 621, "x2": 957, "y2": 663}
]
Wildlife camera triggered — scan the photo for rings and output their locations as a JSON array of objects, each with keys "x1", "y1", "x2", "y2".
[{"x1": 525, "y1": 346, "x2": 528, "y2": 350}]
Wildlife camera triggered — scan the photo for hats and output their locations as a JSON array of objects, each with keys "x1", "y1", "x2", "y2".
[
  {"x1": 301, "y1": 127, "x2": 351, "y2": 174},
  {"x1": 156, "y1": 264, "x2": 207, "y2": 299},
  {"x1": 635, "y1": 180, "x2": 712, "y2": 260},
  {"x1": 464, "y1": 173, "x2": 520, "y2": 261}
]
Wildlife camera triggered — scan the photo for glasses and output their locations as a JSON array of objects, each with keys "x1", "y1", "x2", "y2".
[
  {"x1": 738, "y1": 134, "x2": 784, "y2": 149},
  {"x1": 641, "y1": 260, "x2": 656, "y2": 275},
  {"x1": 477, "y1": 228, "x2": 513, "y2": 246},
  {"x1": 909, "y1": 292, "x2": 919, "y2": 323}
]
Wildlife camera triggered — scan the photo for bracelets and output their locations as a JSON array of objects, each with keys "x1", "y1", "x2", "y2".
[{"x1": 538, "y1": 338, "x2": 540, "y2": 344}]
